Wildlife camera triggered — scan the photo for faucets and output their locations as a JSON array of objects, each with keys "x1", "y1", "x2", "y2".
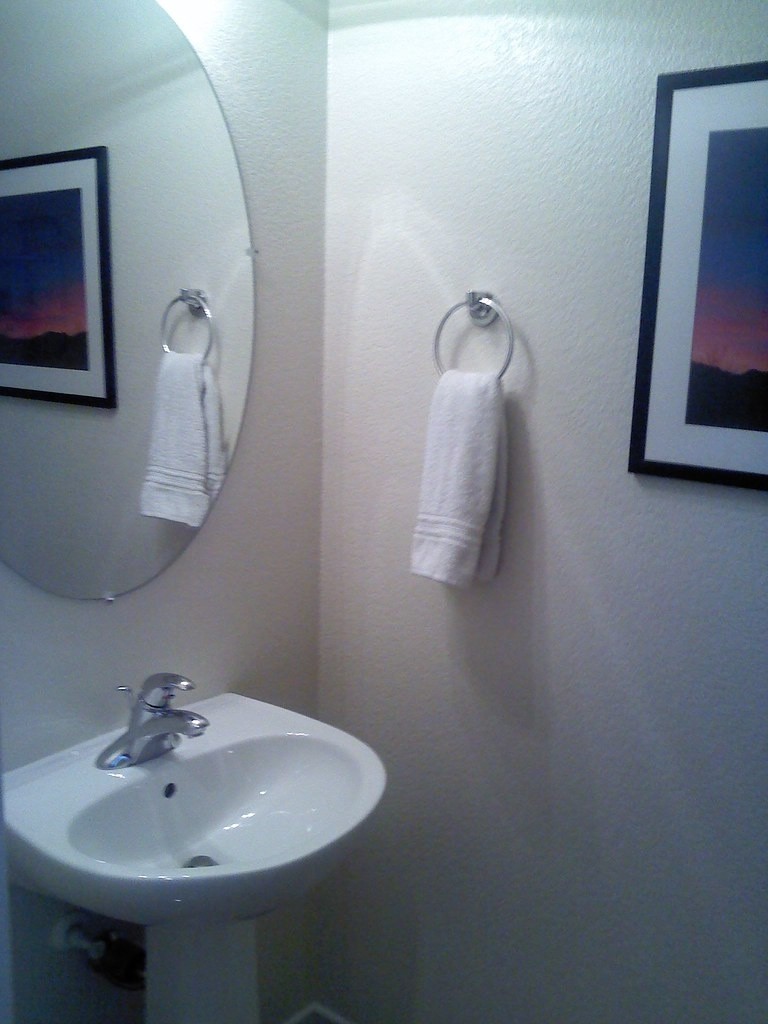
[{"x1": 95, "y1": 671, "x2": 208, "y2": 770}]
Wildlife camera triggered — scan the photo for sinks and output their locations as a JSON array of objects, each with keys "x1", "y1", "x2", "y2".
[{"x1": 2, "y1": 689, "x2": 389, "y2": 928}]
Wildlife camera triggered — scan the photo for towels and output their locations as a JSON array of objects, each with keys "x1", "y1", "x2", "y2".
[
  {"x1": 408, "y1": 368, "x2": 509, "y2": 588},
  {"x1": 138, "y1": 350, "x2": 227, "y2": 527}
]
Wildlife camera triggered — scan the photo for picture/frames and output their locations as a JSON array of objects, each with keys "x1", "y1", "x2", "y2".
[
  {"x1": 627, "y1": 61, "x2": 768, "y2": 491},
  {"x1": 1, "y1": 143, "x2": 118, "y2": 411}
]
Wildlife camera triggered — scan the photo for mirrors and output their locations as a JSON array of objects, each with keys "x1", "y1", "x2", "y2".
[{"x1": 1, "y1": 1, "x2": 258, "y2": 599}]
